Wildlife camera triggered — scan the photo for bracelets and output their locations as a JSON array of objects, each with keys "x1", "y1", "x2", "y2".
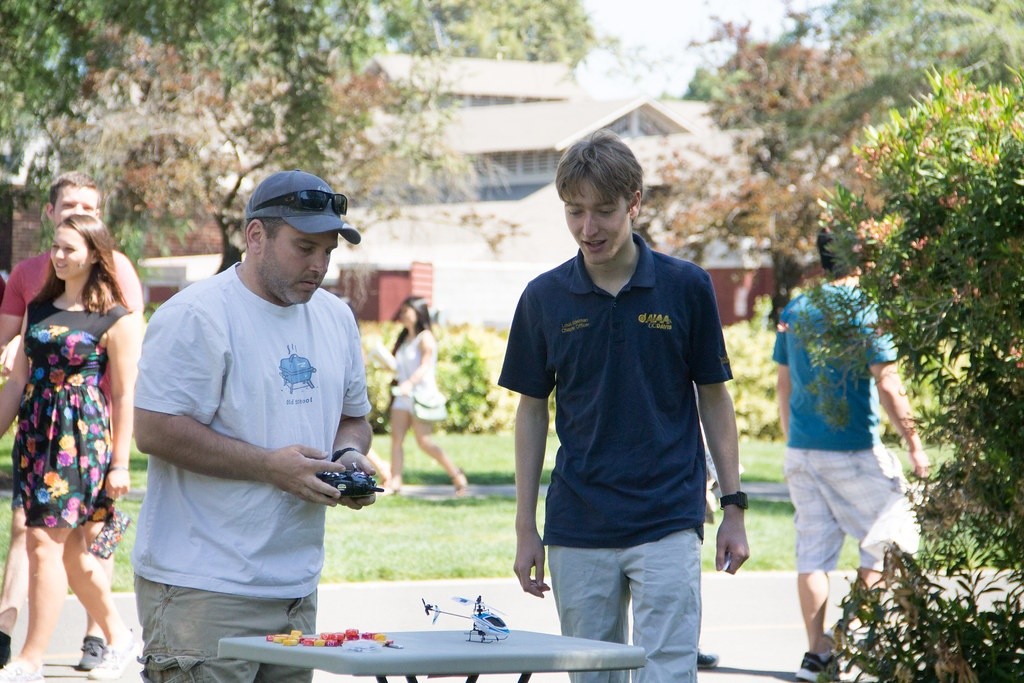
[
  {"x1": 330, "y1": 447, "x2": 363, "y2": 463},
  {"x1": 108, "y1": 467, "x2": 129, "y2": 472}
]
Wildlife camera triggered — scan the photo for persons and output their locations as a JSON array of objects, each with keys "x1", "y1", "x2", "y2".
[
  {"x1": 0, "y1": 173, "x2": 140, "y2": 683},
  {"x1": 772, "y1": 230, "x2": 930, "y2": 683},
  {"x1": 498, "y1": 130, "x2": 751, "y2": 683},
  {"x1": 132, "y1": 171, "x2": 376, "y2": 683},
  {"x1": 364, "y1": 293, "x2": 470, "y2": 498}
]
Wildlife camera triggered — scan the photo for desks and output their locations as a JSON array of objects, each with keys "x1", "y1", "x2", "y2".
[{"x1": 216, "y1": 629, "x2": 647, "y2": 683}]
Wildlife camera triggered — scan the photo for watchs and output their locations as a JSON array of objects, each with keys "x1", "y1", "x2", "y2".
[{"x1": 720, "y1": 490, "x2": 748, "y2": 509}]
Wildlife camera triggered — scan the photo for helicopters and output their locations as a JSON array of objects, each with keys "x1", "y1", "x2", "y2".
[{"x1": 422, "y1": 595, "x2": 511, "y2": 644}]
